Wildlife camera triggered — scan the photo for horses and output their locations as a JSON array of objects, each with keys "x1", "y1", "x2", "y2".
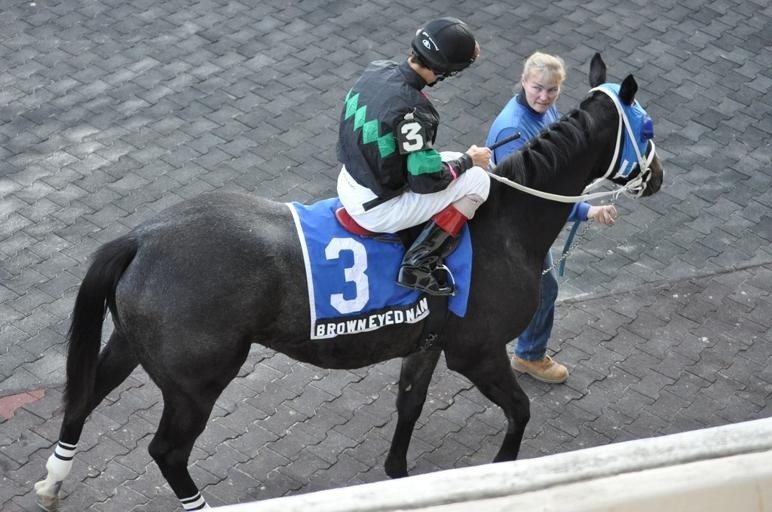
[{"x1": 33, "y1": 52, "x2": 664, "y2": 512}]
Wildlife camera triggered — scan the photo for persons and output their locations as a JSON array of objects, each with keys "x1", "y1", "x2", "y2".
[
  {"x1": 334, "y1": 17, "x2": 494, "y2": 299},
  {"x1": 484, "y1": 51, "x2": 618, "y2": 386}
]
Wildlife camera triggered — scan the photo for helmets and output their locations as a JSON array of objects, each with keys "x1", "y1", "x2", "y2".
[{"x1": 411, "y1": 17, "x2": 480, "y2": 71}]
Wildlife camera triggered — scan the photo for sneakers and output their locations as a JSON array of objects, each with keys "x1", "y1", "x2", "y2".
[{"x1": 510, "y1": 354, "x2": 569, "y2": 384}]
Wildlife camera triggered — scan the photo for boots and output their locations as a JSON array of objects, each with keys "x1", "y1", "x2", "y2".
[{"x1": 394, "y1": 203, "x2": 469, "y2": 296}]
